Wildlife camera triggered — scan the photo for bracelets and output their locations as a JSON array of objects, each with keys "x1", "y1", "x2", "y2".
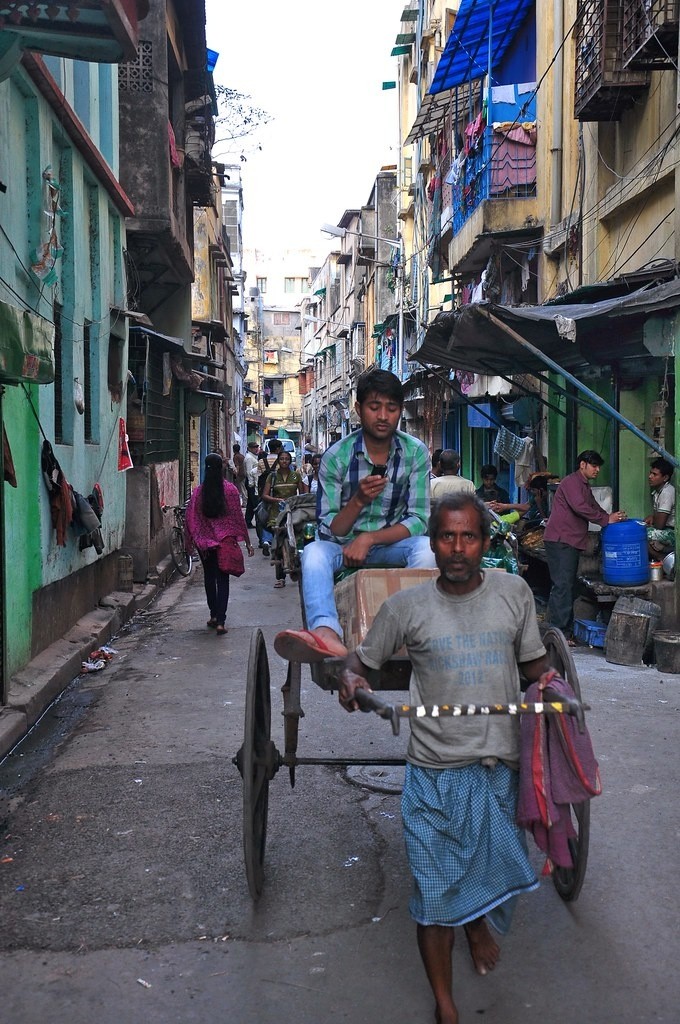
[{"x1": 245, "y1": 543, "x2": 253, "y2": 548}]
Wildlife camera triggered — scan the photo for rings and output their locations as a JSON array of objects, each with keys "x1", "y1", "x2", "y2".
[{"x1": 371, "y1": 488, "x2": 374, "y2": 490}]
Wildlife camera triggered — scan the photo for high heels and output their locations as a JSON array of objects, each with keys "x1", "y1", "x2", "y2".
[
  {"x1": 217, "y1": 625, "x2": 228, "y2": 634},
  {"x1": 206, "y1": 619, "x2": 217, "y2": 627}
]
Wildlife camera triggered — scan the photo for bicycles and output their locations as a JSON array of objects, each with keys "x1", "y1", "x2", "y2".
[{"x1": 161, "y1": 500, "x2": 197, "y2": 577}]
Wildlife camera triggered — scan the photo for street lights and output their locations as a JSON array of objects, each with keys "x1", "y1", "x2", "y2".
[
  {"x1": 303, "y1": 313, "x2": 354, "y2": 433},
  {"x1": 318, "y1": 223, "x2": 404, "y2": 432},
  {"x1": 279, "y1": 347, "x2": 330, "y2": 447}
]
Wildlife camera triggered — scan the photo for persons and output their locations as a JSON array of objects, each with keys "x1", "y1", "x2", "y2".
[
  {"x1": 543, "y1": 449, "x2": 629, "y2": 647},
  {"x1": 273, "y1": 368, "x2": 438, "y2": 664},
  {"x1": 337, "y1": 491, "x2": 564, "y2": 1024},
  {"x1": 641, "y1": 458, "x2": 676, "y2": 561},
  {"x1": 184, "y1": 452, "x2": 254, "y2": 634},
  {"x1": 214, "y1": 438, "x2": 336, "y2": 588},
  {"x1": 430, "y1": 448, "x2": 547, "y2": 527}
]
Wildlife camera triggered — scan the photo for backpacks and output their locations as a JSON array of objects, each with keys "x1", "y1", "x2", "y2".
[{"x1": 257, "y1": 457, "x2": 279, "y2": 497}]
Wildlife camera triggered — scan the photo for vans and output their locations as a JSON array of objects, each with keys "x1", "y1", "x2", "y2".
[{"x1": 262, "y1": 439, "x2": 297, "y2": 470}]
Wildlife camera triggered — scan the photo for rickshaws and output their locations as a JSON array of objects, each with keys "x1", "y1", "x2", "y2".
[
  {"x1": 270, "y1": 493, "x2": 324, "y2": 580},
  {"x1": 230, "y1": 488, "x2": 599, "y2": 905}
]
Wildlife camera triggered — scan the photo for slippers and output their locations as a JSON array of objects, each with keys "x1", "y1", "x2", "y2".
[{"x1": 274, "y1": 628, "x2": 339, "y2": 662}]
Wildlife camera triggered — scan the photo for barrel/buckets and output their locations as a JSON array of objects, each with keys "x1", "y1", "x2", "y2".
[
  {"x1": 605, "y1": 595, "x2": 660, "y2": 664},
  {"x1": 598, "y1": 519, "x2": 650, "y2": 586},
  {"x1": 649, "y1": 562, "x2": 662, "y2": 581},
  {"x1": 652, "y1": 630, "x2": 680, "y2": 673}
]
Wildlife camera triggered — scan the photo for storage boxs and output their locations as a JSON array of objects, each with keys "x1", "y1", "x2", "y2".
[
  {"x1": 573, "y1": 620, "x2": 608, "y2": 647},
  {"x1": 333, "y1": 568, "x2": 507, "y2": 656}
]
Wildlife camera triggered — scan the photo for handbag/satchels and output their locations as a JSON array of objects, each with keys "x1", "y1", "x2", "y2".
[
  {"x1": 217, "y1": 536, "x2": 245, "y2": 578},
  {"x1": 253, "y1": 473, "x2": 275, "y2": 523}
]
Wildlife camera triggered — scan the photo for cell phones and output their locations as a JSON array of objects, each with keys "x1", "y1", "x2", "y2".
[{"x1": 371, "y1": 465, "x2": 387, "y2": 478}]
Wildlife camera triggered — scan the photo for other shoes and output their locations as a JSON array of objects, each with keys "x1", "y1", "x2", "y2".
[
  {"x1": 274, "y1": 580, "x2": 285, "y2": 588},
  {"x1": 566, "y1": 639, "x2": 576, "y2": 647},
  {"x1": 249, "y1": 526, "x2": 256, "y2": 529},
  {"x1": 262, "y1": 541, "x2": 270, "y2": 556}
]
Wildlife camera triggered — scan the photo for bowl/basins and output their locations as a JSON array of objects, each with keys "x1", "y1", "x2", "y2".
[{"x1": 663, "y1": 551, "x2": 675, "y2": 575}]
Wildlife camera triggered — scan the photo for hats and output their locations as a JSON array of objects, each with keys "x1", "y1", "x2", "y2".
[{"x1": 248, "y1": 442, "x2": 259, "y2": 448}]
